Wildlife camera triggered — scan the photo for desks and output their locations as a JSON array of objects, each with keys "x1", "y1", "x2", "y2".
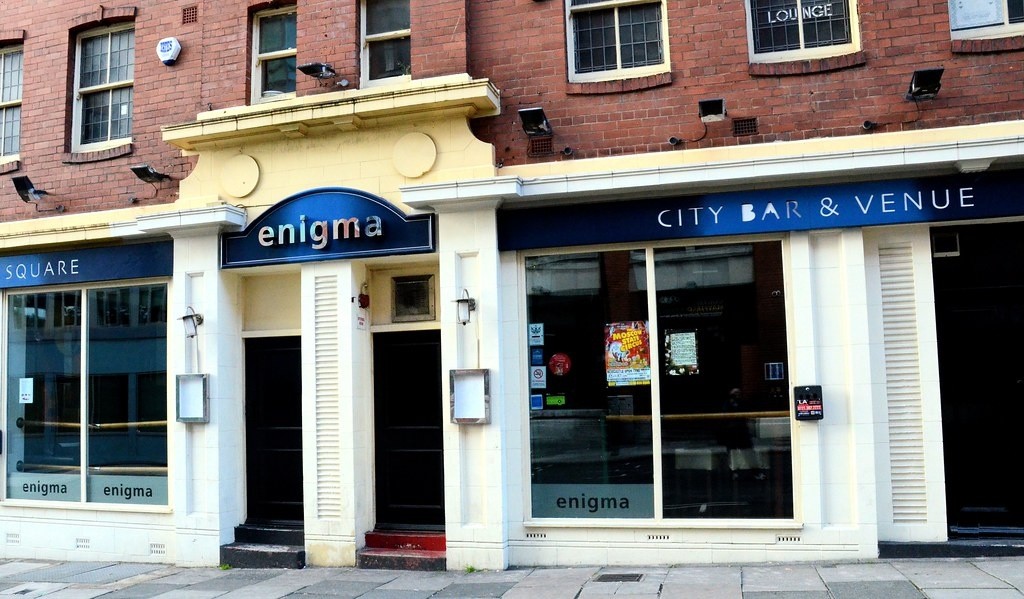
[{"x1": 748, "y1": 416, "x2": 790, "y2": 518}]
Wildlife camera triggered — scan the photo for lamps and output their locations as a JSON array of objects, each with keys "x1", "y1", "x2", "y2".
[
  {"x1": 452, "y1": 288, "x2": 476, "y2": 326},
  {"x1": 175, "y1": 306, "x2": 203, "y2": 339},
  {"x1": 698, "y1": 97, "x2": 727, "y2": 123},
  {"x1": 517, "y1": 107, "x2": 554, "y2": 138},
  {"x1": 11, "y1": 176, "x2": 49, "y2": 204},
  {"x1": 297, "y1": 61, "x2": 340, "y2": 79},
  {"x1": 130, "y1": 165, "x2": 172, "y2": 183},
  {"x1": 906, "y1": 66, "x2": 946, "y2": 100}
]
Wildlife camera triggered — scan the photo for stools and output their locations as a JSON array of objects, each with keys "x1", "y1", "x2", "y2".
[{"x1": 669, "y1": 447, "x2": 772, "y2": 518}]
